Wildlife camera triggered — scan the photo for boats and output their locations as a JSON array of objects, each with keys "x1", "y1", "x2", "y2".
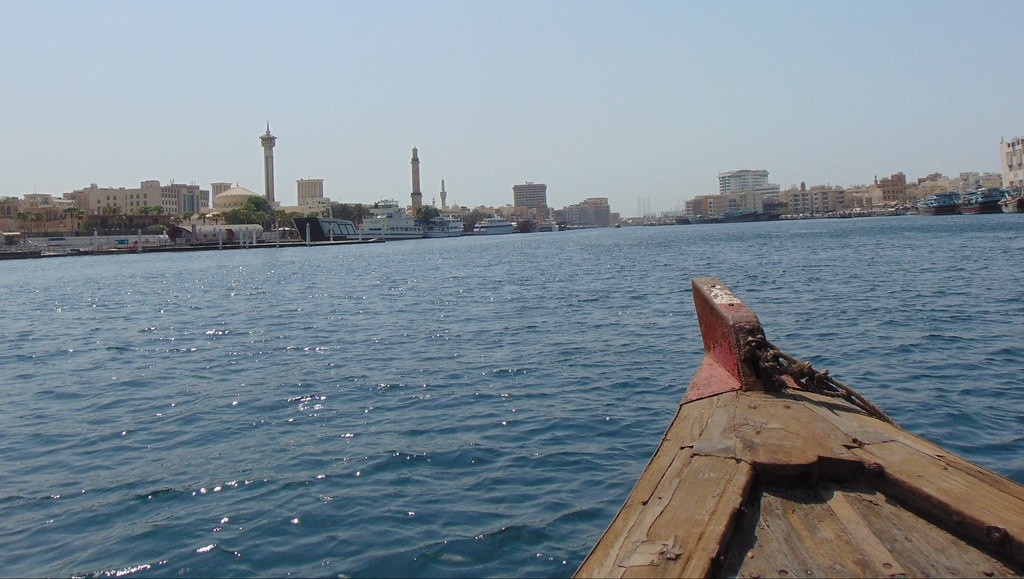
[
  {"x1": 916, "y1": 184, "x2": 1024, "y2": 216},
  {"x1": 674, "y1": 210, "x2": 782, "y2": 226},
  {"x1": 473, "y1": 216, "x2": 515, "y2": 238},
  {"x1": 358, "y1": 199, "x2": 465, "y2": 241}
]
